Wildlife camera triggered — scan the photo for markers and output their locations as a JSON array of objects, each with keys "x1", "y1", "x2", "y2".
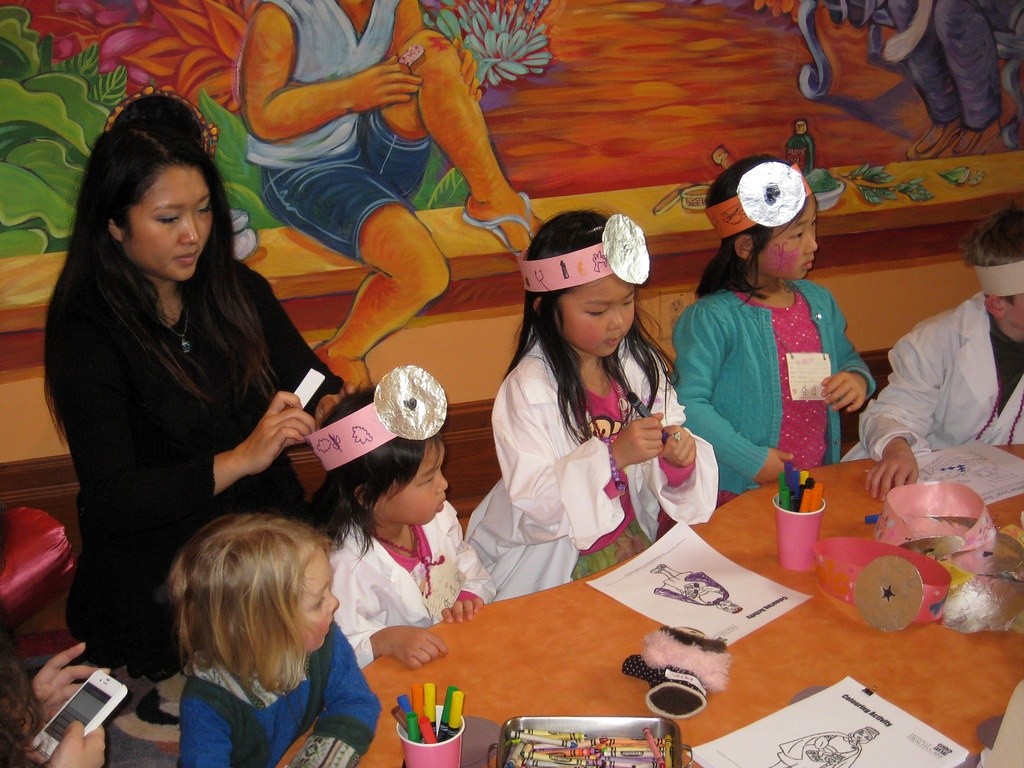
[
  {"x1": 866, "y1": 513, "x2": 879, "y2": 523},
  {"x1": 391, "y1": 684, "x2": 465, "y2": 744},
  {"x1": 777, "y1": 460, "x2": 825, "y2": 514},
  {"x1": 628, "y1": 390, "x2": 669, "y2": 444}
]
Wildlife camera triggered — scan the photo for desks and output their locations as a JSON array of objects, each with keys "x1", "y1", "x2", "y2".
[{"x1": 278, "y1": 440, "x2": 1024, "y2": 768}]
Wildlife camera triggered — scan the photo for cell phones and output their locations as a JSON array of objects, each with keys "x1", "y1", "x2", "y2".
[{"x1": 32, "y1": 670, "x2": 129, "y2": 759}]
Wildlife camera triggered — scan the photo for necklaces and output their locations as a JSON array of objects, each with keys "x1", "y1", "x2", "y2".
[{"x1": 157, "y1": 292, "x2": 191, "y2": 353}]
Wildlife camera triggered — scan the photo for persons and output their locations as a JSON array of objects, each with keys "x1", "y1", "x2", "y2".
[
  {"x1": 841, "y1": 203, "x2": 1024, "y2": 502},
  {"x1": 669, "y1": 154, "x2": 878, "y2": 510},
  {"x1": 168, "y1": 511, "x2": 382, "y2": 768},
  {"x1": 0, "y1": 595, "x2": 111, "y2": 768},
  {"x1": 43, "y1": 120, "x2": 374, "y2": 675},
  {"x1": 305, "y1": 386, "x2": 497, "y2": 672},
  {"x1": 463, "y1": 207, "x2": 718, "y2": 604}
]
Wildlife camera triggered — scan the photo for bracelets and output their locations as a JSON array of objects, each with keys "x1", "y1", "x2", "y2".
[{"x1": 605, "y1": 436, "x2": 627, "y2": 493}]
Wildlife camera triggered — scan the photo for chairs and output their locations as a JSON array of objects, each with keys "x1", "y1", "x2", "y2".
[{"x1": 0, "y1": 503, "x2": 78, "y2": 629}]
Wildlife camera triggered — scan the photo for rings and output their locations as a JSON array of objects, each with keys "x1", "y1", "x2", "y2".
[{"x1": 669, "y1": 432, "x2": 682, "y2": 441}]
